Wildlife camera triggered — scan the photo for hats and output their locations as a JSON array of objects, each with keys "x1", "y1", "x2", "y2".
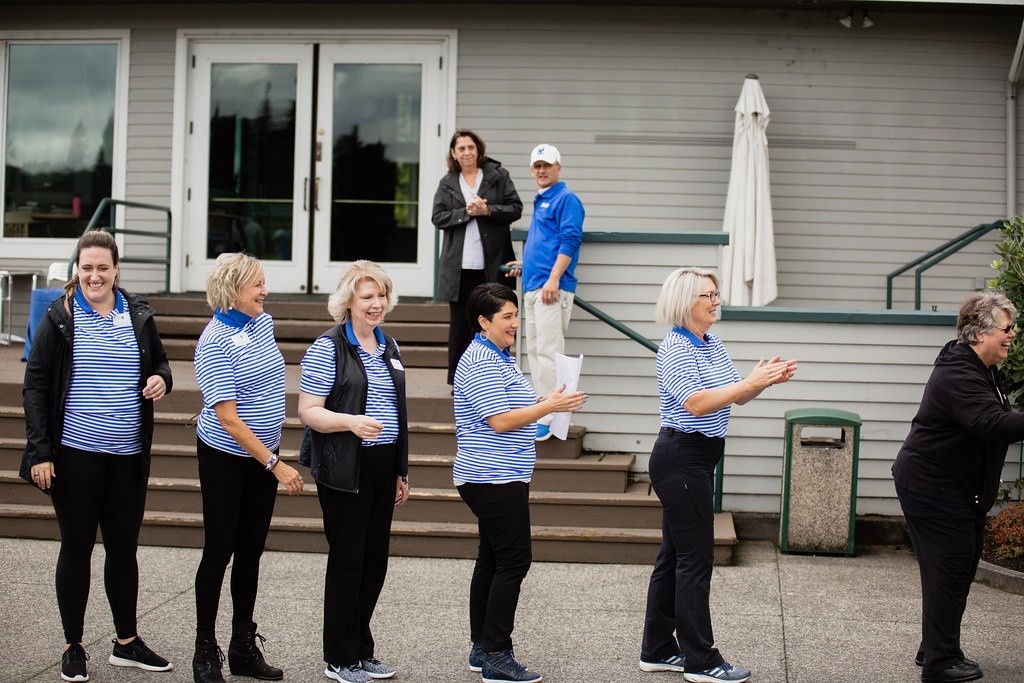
[{"x1": 531, "y1": 144, "x2": 561, "y2": 167}]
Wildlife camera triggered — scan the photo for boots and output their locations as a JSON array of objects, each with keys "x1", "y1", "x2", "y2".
[
  {"x1": 228, "y1": 619, "x2": 284, "y2": 680},
  {"x1": 192, "y1": 636, "x2": 227, "y2": 683}
]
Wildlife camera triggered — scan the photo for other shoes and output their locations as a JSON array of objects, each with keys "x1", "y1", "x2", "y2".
[{"x1": 535, "y1": 422, "x2": 553, "y2": 441}]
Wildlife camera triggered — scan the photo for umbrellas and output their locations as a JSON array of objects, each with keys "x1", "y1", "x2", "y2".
[{"x1": 720, "y1": 73, "x2": 783, "y2": 308}]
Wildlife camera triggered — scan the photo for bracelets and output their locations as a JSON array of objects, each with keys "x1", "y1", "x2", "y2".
[
  {"x1": 265, "y1": 454, "x2": 277, "y2": 470},
  {"x1": 270, "y1": 456, "x2": 280, "y2": 471}
]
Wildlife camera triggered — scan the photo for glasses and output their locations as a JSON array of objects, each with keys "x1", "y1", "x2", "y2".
[
  {"x1": 995, "y1": 324, "x2": 1015, "y2": 333},
  {"x1": 699, "y1": 290, "x2": 720, "y2": 302},
  {"x1": 534, "y1": 164, "x2": 551, "y2": 169}
]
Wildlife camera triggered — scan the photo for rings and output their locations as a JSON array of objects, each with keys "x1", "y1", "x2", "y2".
[
  {"x1": 34, "y1": 472, "x2": 39, "y2": 476},
  {"x1": 299, "y1": 476, "x2": 303, "y2": 481},
  {"x1": 769, "y1": 373, "x2": 773, "y2": 377}
]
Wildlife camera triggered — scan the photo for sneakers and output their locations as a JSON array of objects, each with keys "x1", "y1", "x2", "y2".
[
  {"x1": 325, "y1": 661, "x2": 375, "y2": 683},
  {"x1": 684, "y1": 659, "x2": 752, "y2": 683},
  {"x1": 468, "y1": 640, "x2": 529, "y2": 672},
  {"x1": 639, "y1": 652, "x2": 686, "y2": 672},
  {"x1": 60, "y1": 642, "x2": 90, "y2": 681},
  {"x1": 481, "y1": 650, "x2": 543, "y2": 683},
  {"x1": 358, "y1": 656, "x2": 397, "y2": 679},
  {"x1": 108, "y1": 636, "x2": 175, "y2": 671}
]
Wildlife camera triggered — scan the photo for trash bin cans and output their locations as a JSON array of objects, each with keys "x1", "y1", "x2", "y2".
[{"x1": 779, "y1": 407, "x2": 862, "y2": 554}]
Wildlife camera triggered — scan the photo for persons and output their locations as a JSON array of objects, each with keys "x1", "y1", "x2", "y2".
[
  {"x1": 890, "y1": 293, "x2": 1024, "y2": 683},
  {"x1": 506, "y1": 144, "x2": 585, "y2": 442},
  {"x1": 637, "y1": 268, "x2": 801, "y2": 683},
  {"x1": 19, "y1": 230, "x2": 175, "y2": 682},
  {"x1": 191, "y1": 253, "x2": 306, "y2": 683},
  {"x1": 431, "y1": 130, "x2": 525, "y2": 396},
  {"x1": 297, "y1": 260, "x2": 410, "y2": 683},
  {"x1": 453, "y1": 282, "x2": 590, "y2": 682}
]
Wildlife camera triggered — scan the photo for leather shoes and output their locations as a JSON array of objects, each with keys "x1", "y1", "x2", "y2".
[
  {"x1": 920, "y1": 661, "x2": 983, "y2": 683},
  {"x1": 914, "y1": 648, "x2": 979, "y2": 670}
]
